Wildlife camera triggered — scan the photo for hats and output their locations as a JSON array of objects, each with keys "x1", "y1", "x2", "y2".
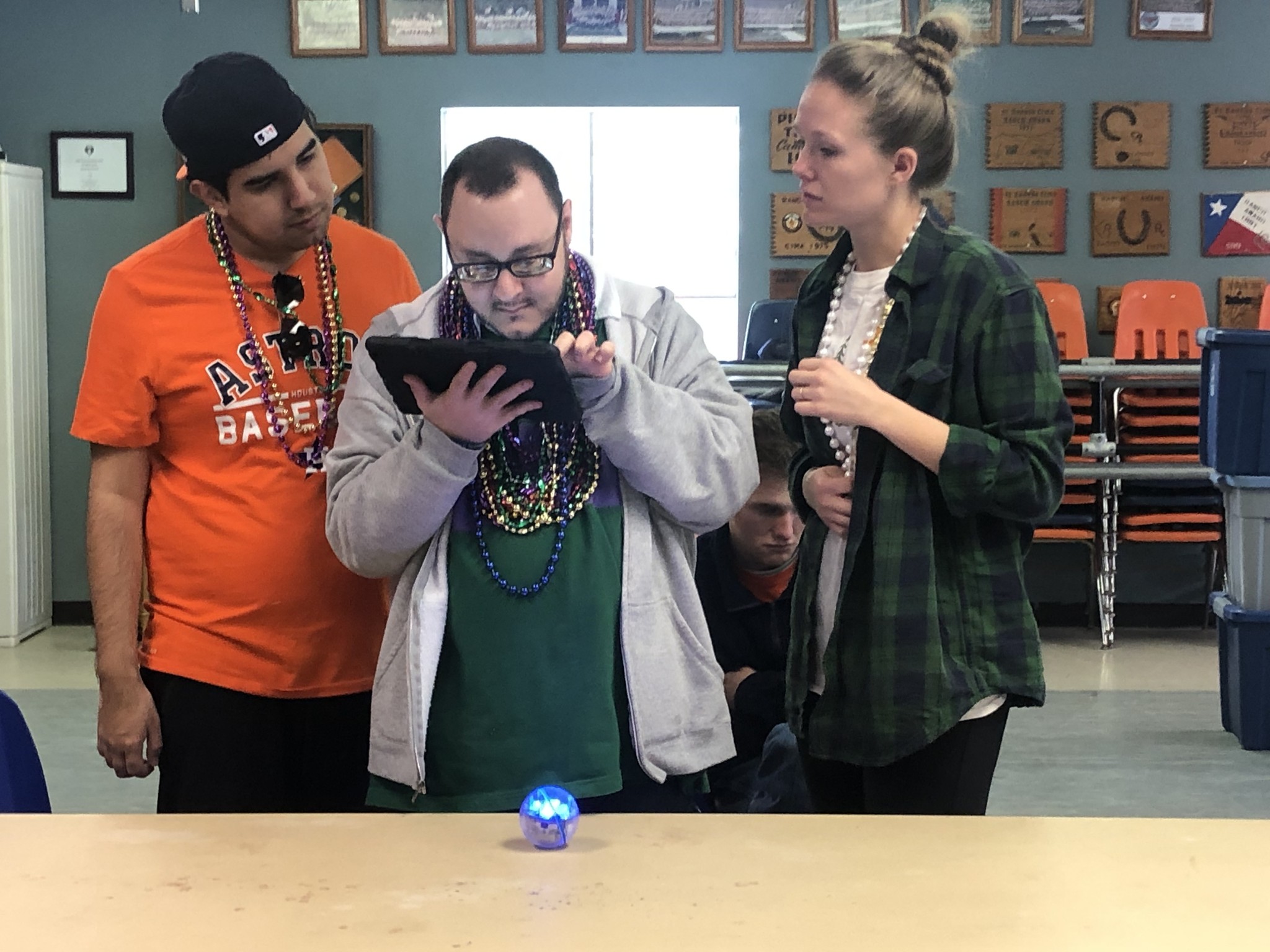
[{"x1": 161, "y1": 52, "x2": 304, "y2": 177}]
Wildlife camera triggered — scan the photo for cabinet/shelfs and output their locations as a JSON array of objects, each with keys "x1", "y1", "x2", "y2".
[{"x1": 0, "y1": 161, "x2": 51, "y2": 646}]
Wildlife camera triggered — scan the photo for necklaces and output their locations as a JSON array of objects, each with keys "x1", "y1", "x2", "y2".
[
  {"x1": 813, "y1": 204, "x2": 928, "y2": 476},
  {"x1": 206, "y1": 209, "x2": 346, "y2": 469},
  {"x1": 438, "y1": 250, "x2": 600, "y2": 598}
]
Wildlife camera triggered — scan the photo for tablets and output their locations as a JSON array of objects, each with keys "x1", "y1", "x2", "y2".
[{"x1": 365, "y1": 336, "x2": 583, "y2": 420}]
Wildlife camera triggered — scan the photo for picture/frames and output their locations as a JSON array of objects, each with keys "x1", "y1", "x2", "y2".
[
  {"x1": 50, "y1": 131, "x2": 134, "y2": 200},
  {"x1": 177, "y1": 122, "x2": 375, "y2": 228},
  {"x1": 1130, "y1": 0, "x2": 1213, "y2": 40},
  {"x1": 290, "y1": 0, "x2": 368, "y2": 58},
  {"x1": 467, "y1": 0, "x2": 545, "y2": 53},
  {"x1": 558, "y1": 0, "x2": 635, "y2": 51},
  {"x1": 378, "y1": 0, "x2": 457, "y2": 54},
  {"x1": 1012, "y1": 0, "x2": 1095, "y2": 47},
  {"x1": 732, "y1": 0, "x2": 815, "y2": 50},
  {"x1": 826, "y1": 0, "x2": 910, "y2": 48},
  {"x1": 642, "y1": 0, "x2": 725, "y2": 52},
  {"x1": 918, "y1": 0, "x2": 1001, "y2": 46}
]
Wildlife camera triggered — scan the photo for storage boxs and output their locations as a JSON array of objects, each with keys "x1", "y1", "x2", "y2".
[
  {"x1": 1208, "y1": 472, "x2": 1270, "y2": 611},
  {"x1": 1209, "y1": 590, "x2": 1270, "y2": 751},
  {"x1": 1198, "y1": 327, "x2": 1270, "y2": 474}
]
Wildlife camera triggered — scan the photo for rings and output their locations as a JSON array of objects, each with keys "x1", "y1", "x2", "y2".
[{"x1": 799, "y1": 387, "x2": 805, "y2": 400}]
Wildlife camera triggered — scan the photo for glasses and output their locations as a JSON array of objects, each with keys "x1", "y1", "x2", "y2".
[{"x1": 444, "y1": 209, "x2": 562, "y2": 283}]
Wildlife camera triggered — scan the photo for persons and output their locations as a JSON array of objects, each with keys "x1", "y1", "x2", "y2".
[
  {"x1": 776, "y1": 15, "x2": 1074, "y2": 815},
  {"x1": 323, "y1": 135, "x2": 760, "y2": 815},
  {"x1": 693, "y1": 408, "x2": 815, "y2": 812},
  {"x1": 69, "y1": 51, "x2": 421, "y2": 814}
]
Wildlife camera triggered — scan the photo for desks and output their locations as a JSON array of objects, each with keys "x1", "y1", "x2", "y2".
[{"x1": 0, "y1": 814, "x2": 1270, "y2": 952}]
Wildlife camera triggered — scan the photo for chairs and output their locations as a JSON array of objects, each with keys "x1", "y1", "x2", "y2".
[{"x1": 745, "y1": 280, "x2": 1223, "y2": 643}]
